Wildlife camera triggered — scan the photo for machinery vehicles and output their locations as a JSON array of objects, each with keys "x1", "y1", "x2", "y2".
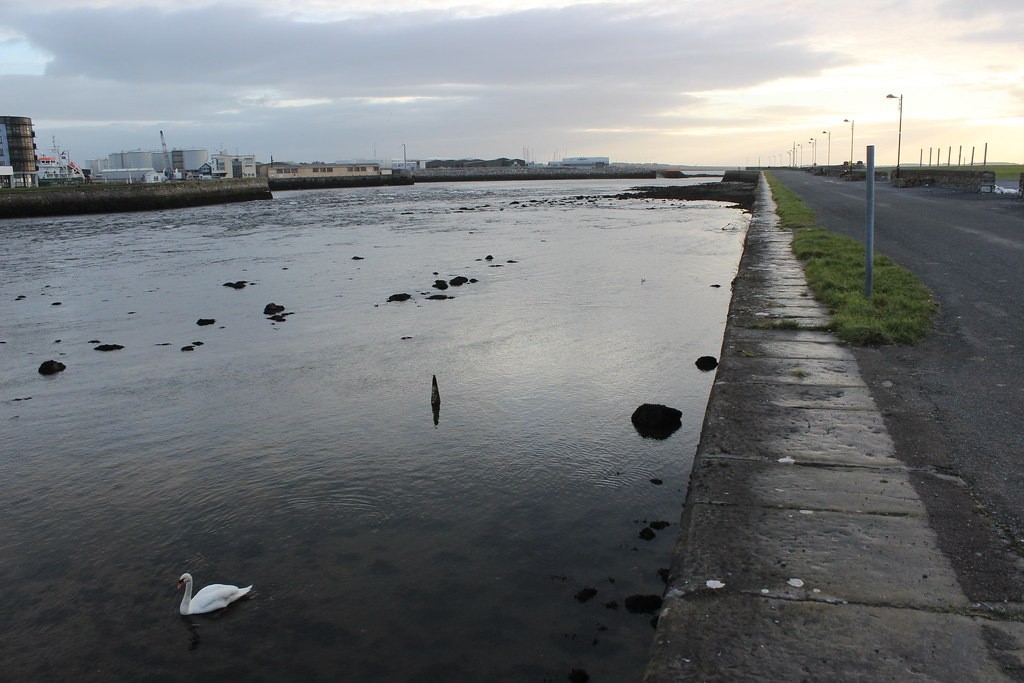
[{"x1": 159, "y1": 130, "x2": 182, "y2": 182}]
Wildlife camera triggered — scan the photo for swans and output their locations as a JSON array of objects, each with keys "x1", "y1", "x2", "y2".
[{"x1": 175, "y1": 572, "x2": 253, "y2": 616}]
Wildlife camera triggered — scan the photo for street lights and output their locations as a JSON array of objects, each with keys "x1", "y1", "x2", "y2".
[
  {"x1": 843, "y1": 119, "x2": 854, "y2": 177},
  {"x1": 823, "y1": 131, "x2": 831, "y2": 176},
  {"x1": 787, "y1": 138, "x2": 817, "y2": 172},
  {"x1": 886, "y1": 94, "x2": 903, "y2": 185}
]
